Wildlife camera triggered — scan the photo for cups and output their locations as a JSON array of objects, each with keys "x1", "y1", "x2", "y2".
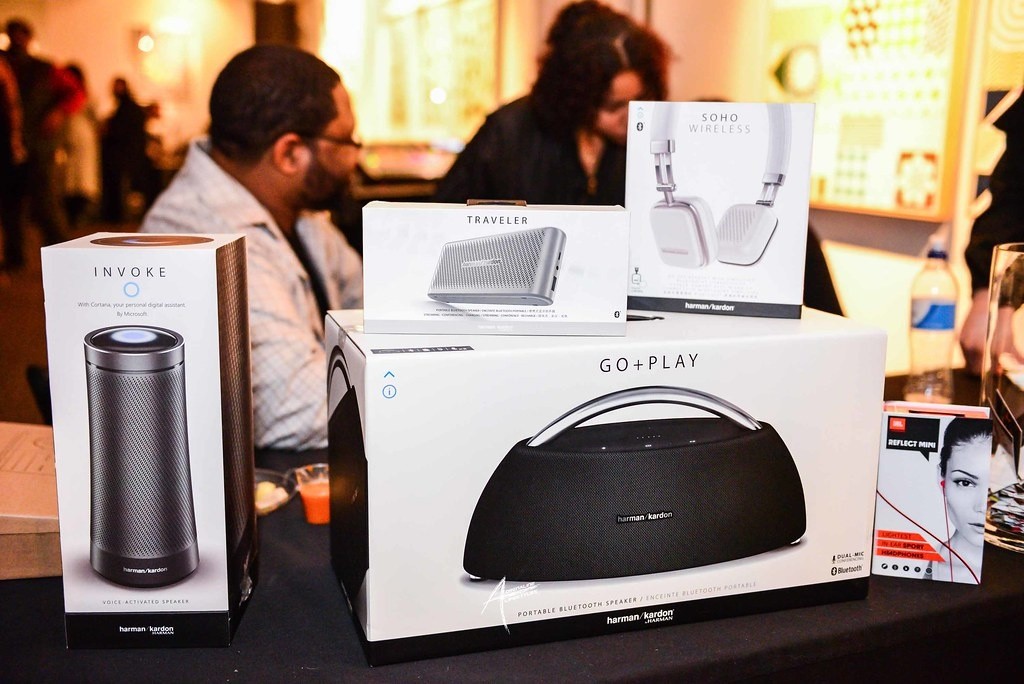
[{"x1": 980, "y1": 242, "x2": 1024, "y2": 556}]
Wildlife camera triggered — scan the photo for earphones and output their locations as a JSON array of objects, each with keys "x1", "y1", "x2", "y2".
[{"x1": 940, "y1": 480, "x2": 946, "y2": 488}]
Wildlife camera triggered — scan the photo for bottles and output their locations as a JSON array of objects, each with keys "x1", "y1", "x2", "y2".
[{"x1": 905, "y1": 249, "x2": 962, "y2": 403}]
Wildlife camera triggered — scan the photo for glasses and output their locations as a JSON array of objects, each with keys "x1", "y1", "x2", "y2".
[{"x1": 274, "y1": 123, "x2": 365, "y2": 157}]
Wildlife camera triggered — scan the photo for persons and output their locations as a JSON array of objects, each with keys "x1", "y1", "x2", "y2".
[
  {"x1": 437, "y1": 0, "x2": 676, "y2": 206},
  {"x1": 140, "y1": 45, "x2": 362, "y2": 450},
  {"x1": 990, "y1": 254, "x2": 1023, "y2": 374},
  {"x1": 921, "y1": 418, "x2": 994, "y2": 584},
  {"x1": 959, "y1": 92, "x2": 1024, "y2": 376},
  {"x1": 0, "y1": 18, "x2": 169, "y2": 274}
]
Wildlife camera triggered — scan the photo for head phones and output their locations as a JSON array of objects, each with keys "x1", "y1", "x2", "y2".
[{"x1": 649, "y1": 101, "x2": 788, "y2": 269}]
